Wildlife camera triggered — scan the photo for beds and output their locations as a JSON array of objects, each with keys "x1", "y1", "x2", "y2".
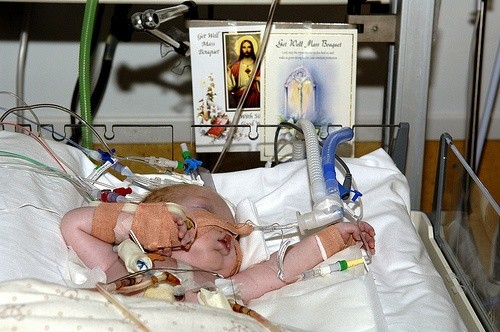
[{"x1": 0, "y1": 122, "x2": 500, "y2": 332}]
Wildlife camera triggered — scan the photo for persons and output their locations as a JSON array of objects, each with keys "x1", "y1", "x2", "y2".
[{"x1": 59, "y1": 184, "x2": 376, "y2": 312}]
